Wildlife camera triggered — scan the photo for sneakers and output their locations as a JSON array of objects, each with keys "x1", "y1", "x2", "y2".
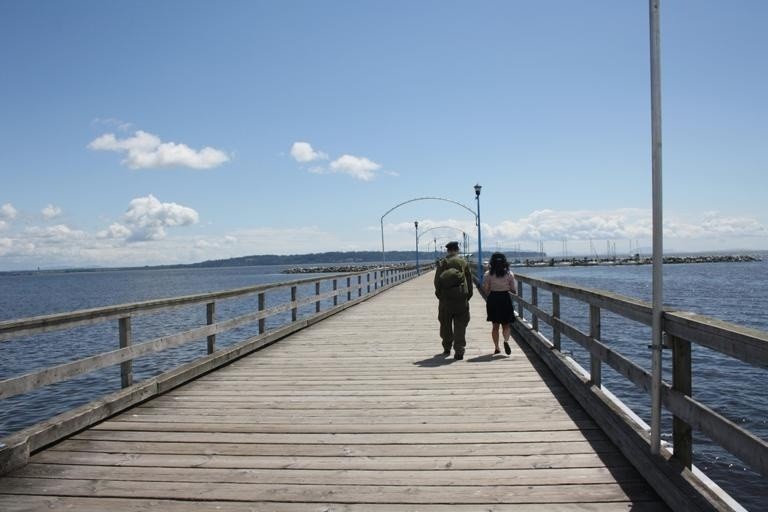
[
  {"x1": 454, "y1": 351, "x2": 463, "y2": 359},
  {"x1": 494, "y1": 349, "x2": 500, "y2": 354},
  {"x1": 503, "y1": 342, "x2": 510, "y2": 355}
]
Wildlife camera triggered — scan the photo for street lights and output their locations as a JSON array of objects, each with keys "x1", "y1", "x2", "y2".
[
  {"x1": 414, "y1": 221, "x2": 420, "y2": 273},
  {"x1": 463, "y1": 231, "x2": 469, "y2": 261},
  {"x1": 433, "y1": 238, "x2": 446, "y2": 254},
  {"x1": 473, "y1": 183, "x2": 483, "y2": 291}
]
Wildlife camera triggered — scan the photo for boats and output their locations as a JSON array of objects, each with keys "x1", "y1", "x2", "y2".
[
  {"x1": 281, "y1": 263, "x2": 391, "y2": 274},
  {"x1": 638, "y1": 254, "x2": 763, "y2": 265}
]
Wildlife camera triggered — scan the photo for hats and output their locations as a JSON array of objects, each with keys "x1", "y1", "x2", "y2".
[{"x1": 445, "y1": 241, "x2": 459, "y2": 248}]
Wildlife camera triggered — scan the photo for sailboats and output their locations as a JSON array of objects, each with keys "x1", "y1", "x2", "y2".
[{"x1": 508, "y1": 239, "x2": 644, "y2": 268}]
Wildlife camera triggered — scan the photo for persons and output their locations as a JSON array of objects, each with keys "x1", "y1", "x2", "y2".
[
  {"x1": 482, "y1": 252, "x2": 518, "y2": 355},
  {"x1": 434, "y1": 241, "x2": 473, "y2": 360}
]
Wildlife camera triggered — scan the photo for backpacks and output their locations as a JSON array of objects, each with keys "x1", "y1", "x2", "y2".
[{"x1": 438, "y1": 258, "x2": 469, "y2": 315}]
